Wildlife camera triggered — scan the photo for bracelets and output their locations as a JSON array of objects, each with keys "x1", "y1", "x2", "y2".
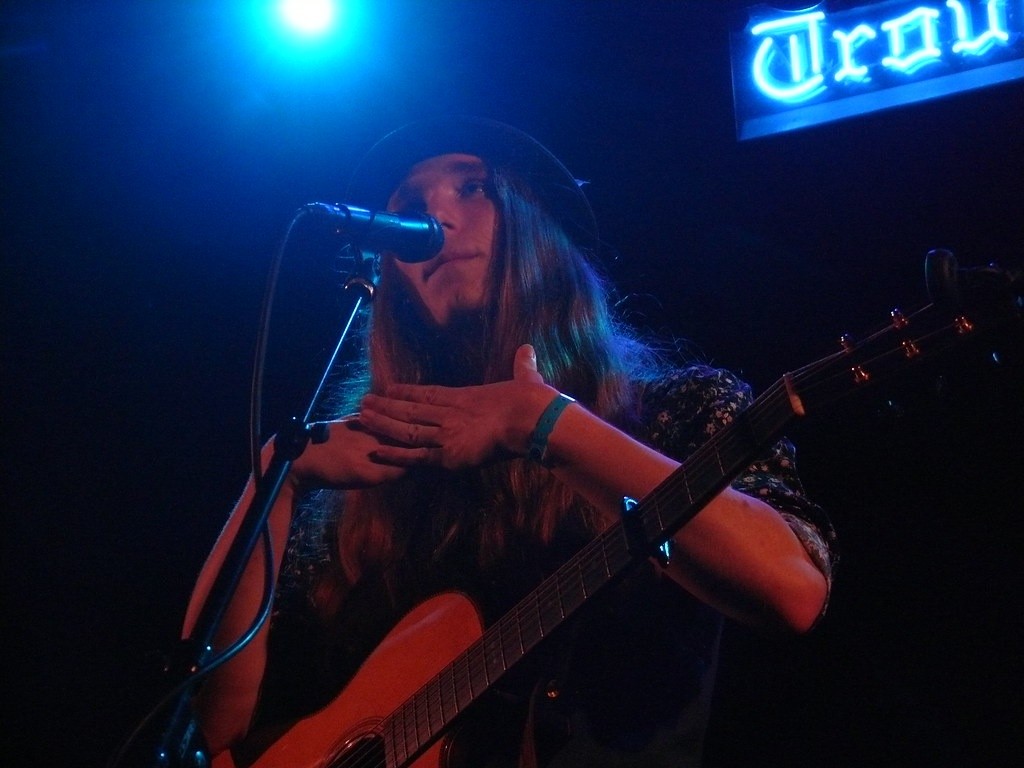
[{"x1": 521, "y1": 393, "x2": 578, "y2": 466}]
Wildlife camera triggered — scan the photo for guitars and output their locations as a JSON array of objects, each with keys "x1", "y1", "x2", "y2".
[{"x1": 205, "y1": 249, "x2": 1015, "y2": 767}]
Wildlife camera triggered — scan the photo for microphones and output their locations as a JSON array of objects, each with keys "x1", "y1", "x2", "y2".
[{"x1": 305, "y1": 201, "x2": 445, "y2": 264}]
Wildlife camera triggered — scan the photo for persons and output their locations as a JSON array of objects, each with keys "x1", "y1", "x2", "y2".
[{"x1": 183, "y1": 113, "x2": 837, "y2": 768}]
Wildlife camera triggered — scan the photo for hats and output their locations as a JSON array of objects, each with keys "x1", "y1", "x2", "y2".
[{"x1": 338, "y1": 112, "x2": 606, "y2": 306}]
